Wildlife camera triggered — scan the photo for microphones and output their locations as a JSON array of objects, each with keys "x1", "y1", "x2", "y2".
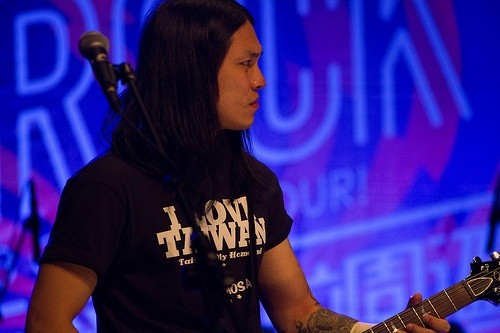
[{"x1": 79, "y1": 31, "x2": 119, "y2": 106}]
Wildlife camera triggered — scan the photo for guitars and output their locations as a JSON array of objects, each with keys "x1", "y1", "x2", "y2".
[{"x1": 362, "y1": 251, "x2": 500, "y2": 333}]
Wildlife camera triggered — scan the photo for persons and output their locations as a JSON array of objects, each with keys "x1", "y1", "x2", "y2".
[{"x1": 25, "y1": 0, "x2": 451, "y2": 333}]
